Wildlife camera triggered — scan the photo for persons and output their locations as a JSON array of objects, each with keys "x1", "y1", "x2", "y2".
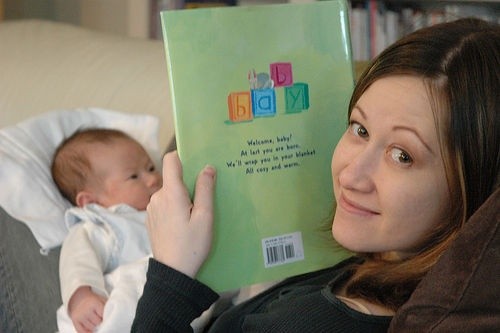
[
  {"x1": 132, "y1": 17, "x2": 499, "y2": 333},
  {"x1": 51, "y1": 123, "x2": 162, "y2": 333}
]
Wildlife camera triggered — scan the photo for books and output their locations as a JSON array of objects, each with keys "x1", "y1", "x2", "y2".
[{"x1": 159, "y1": 0, "x2": 357, "y2": 294}]
